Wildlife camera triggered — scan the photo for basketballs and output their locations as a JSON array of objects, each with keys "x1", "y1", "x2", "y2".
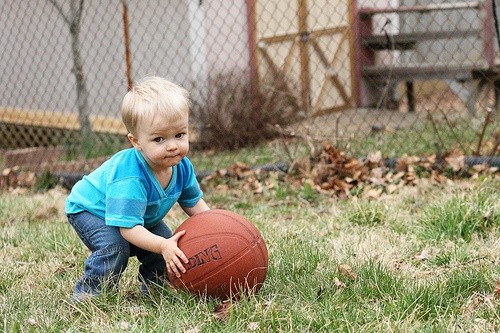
[{"x1": 162, "y1": 209, "x2": 268, "y2": 305}]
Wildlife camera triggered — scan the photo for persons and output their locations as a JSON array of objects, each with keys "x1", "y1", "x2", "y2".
[{"x1": 64, "y1": 76, "x2": 213, "y2": 306}]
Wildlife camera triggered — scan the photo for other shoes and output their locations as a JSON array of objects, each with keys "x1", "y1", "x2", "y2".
[
  {"x1": 73, "y1": 283, "x2": 115, "y2": 299},
  {"x1": 142, "y1": 283, "x2": 171, "y2": 295}
]
